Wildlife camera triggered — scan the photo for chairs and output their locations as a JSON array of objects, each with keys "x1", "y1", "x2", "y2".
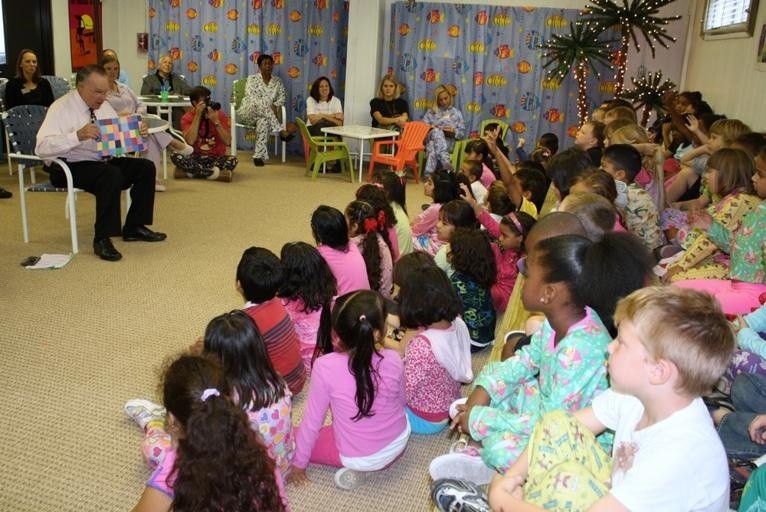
[
  {"x1": 42, "y1": 75, "x2": 71, "y2": 100},
  {"x1": 368, "y1": 121, "x2": 430, "y2": 183},
  {"x1": 42, "y1": 76, "x2": 161, "y2": 220},
  {"x1": 418, "y1": 139, "x2": 462, "y2": 178},
  {"x1": 0, "y1": 85, "x2": 36, "y2": 184},
  {"x1": 2, "y1": 104, "x2": 78, "y2": 254},
  {"x1": 230, "y1": 78, "x2": 287, "y2": 162},
  {"x1": 295, "y1": 115, "x2": 355, "y2": 182},
  {"x1": 462, "y1": 120, "x2": 511, "y2": 172}
]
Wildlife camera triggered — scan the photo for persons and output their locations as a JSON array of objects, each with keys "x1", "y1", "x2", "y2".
[
  {"x1": 305, "y1": 76, "x2": 344, "y2": 172},
  {"x1": 311, "y1": 205, "x2": 371, "y2": 296},
  {"x1": 189, "y1": 247, "x2": 306, "y2": 401},
  {"x1": 35, "y1": 64, "x2": 167, "y2": 262},
  {"x1": 170, "y1": 86, "x2": 239, "y2": 182},
  {"x1": 344, "y1": 199, "x2": 393, "y2": 298},
  {"x1": 398, "y1": 263, "x2": 473, "y2": 434},
  {"x1": 99, "y1": 55, "x2": 194, "y2": 192},
  {"x1": 370, "y1": 75, "x2": 409, "y2": 153},
  {"x1": 5, "y1": 48, "x2": 55, "y2": 173},
  {"x1": 130, "y1": 351, "x2": 288, "y2": 512},
  {"x1": 522, "y1": 99, "x2": 664, "y2": 253},
  {"x1": 434, "y1": 199, "x2": 480, "y2": 280},
  {"x1": 460, "y1": 181, "x2": 538, "y2": 314},
  {"x1": 276, "y1": 242, "x2": 339, "y2": 379},
  {"x1": 125, "y1": 309, "x2": 296, "y2": 483},
  {"x1": 446, "y1": 226, "x2": 497, "y2": 353},
  {"x1": 237, "y1": 55, "x2": 295, "y2": 166},
  {"x1": 450, "y1": 233, "x2": 656, "y2": 474},
  {"x1": 141, "y1": 55, "x2": 194, "y2": 130},
  {"x1": 410, "y1": 167, "x2": 462, "y2": 256},
  {"x1": 284, "y1": 290, "x2": 411, "y2": 489},
  {"x1": 650, "y1": 91, "x2": 765, "y2": 512},
  {"x1": 461, "y1": 122, "x2": 559, "y2": 224},
  {"x1": 375, "y1": 172, "x2": 413, "y2": 258},
  {"x1": 420, "y1": 84, "x2": 465, "y2": 181},
  {"x1": 428, "y1": 286, "x2": 730, "y2": 512},
  {"x1": 356, "y1": 184, "x2": 400, "y2": 263}
]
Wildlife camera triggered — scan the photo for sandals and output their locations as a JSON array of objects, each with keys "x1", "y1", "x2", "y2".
[
  {"x1": 155, "y1": 182, "x2": 165, "y2": 191},
  {"x1": 169, "y1": 140, "x2": 193, "y2": 155}
]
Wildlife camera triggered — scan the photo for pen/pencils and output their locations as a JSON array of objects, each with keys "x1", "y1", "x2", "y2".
[{"x1": 160, "y1": 85, "x2": 172, "y2": 96}]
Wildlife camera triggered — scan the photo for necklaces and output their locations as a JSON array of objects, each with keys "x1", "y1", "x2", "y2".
[{"x1": 110, "y1": 84, "x2": 117, "y2": 89}]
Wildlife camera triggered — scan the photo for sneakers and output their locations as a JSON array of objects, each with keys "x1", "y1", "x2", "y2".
[
  {"x1": 429, "y1": 448, "x2": 496, "y2": 485},
  {"x1": 209, "y1": 167, "x2": 231, "y2": 182},
  {"x1": 173, "y1": 166, "x2": 192, "y2": 178},
  {"x1": 335, "y1": 468, "x2": 371, "y2": 489},
  {"x1": 430, "y1": 478, "x2": 491, "y2": 512},
  {"x1": 125, "y1": 400, "x2": 170, "y2": 430}
]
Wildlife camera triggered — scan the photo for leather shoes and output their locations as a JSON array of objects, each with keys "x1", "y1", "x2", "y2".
[
  {"x1": 279, "y1": 132, "x2": 294, "y2": 141},
  {"x1": 253, "y1": 157, "x2": 265, "y2": 167},
  {"x1": 89, "y1": 108, "x2": 97, "y2": 126},
  {"x1": 92, "y1": 237, "x2": 122, "y2": 261},
  {"x1": 122, "y1": 225, "x2": 166, "y2": 242}
]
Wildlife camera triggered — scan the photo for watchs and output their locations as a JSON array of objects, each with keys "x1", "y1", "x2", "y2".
[{"x1": 215, "y1": 122, "x2": 221, "y2": 128}]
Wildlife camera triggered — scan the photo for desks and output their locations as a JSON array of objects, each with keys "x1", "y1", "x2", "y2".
[
  {"x1": 137, "y1": 94, "x2": 192, "y2": 180},
  {"x1": 320, "y1": 124, "x2": 400, "y2": 182}
]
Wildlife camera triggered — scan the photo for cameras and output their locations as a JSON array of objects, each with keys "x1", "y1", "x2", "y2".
[
  {"x1": 660, "y1": 114, "x2": 699, "y2": 124},
  {"x1": 204, "y1": 99, "x2": 221, "y2": 111}
]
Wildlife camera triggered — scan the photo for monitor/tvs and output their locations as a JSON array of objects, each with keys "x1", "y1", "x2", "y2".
[{"x1": 703, "y1": 0, "x2": 753, "y2": 34}]
